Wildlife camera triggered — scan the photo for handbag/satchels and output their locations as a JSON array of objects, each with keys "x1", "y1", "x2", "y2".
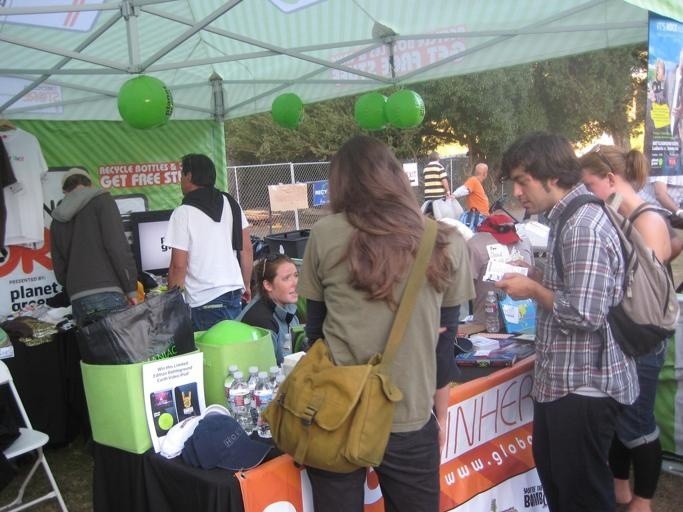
[{"x1": 258, "y1": 217, "x2": 438, "y2": 468}]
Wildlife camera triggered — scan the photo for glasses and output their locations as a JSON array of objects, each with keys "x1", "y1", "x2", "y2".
[
  {"x1": 262, "y1": 253, "x2": 286, "y2": 278},
  {"x1": 485, "y1": 221, "x2": 516, "y2": 233},
  {"x1": 590, "y1": 144, "x2": 616, "y2": 173}
]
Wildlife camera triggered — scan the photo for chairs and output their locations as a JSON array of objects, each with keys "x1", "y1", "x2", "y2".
[{"x1": 0, "y1": 360, "x2": 70, "y2": 512}]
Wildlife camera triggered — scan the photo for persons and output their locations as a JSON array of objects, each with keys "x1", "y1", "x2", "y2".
[
  {"x1": 242, "y1": 255, "x2": 308, "y2": 367},
  {"x1": 580, "y1": 143, "x2": 673, "y2": 511},
  {"x1": 51, "y1": 167, "x2": 139, "y2": 324},
  {"x1": 495, "y1": 133, "x2": 639, "y2": 512},
  {"x1": 422, "y1": 150, "x2": 452, "y2": 201},
  {"x1": 449, "y1": 163, "x2": 489, "y2": 218},
  {"x1": 651, "y1": 180, "x2": 682, "y2": 459},
  {"x1": 161, "y1": 153, "x2": 254, "y2": 332},
  {"x1": 295, "y1": 134, "x2": 477, "y2": 511}
]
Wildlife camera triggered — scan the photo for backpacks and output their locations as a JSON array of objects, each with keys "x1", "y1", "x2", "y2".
[{"x1": 554, "y1": 194, "x2": 679, "y2": 354}]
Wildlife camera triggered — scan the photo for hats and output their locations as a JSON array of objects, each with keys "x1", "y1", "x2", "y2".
[
  {"x1": 182, "y1": 414, "x2": 272, "y2": 470},
  {"x1": 161, "y1": 403, "x2": 230, "y2": 459},
  {"x1": 62, "y1": 168, "x2": 93, "y2": 191},
  {"x1": 478, "y1": 215, "x2": 520, "y2": 245}
]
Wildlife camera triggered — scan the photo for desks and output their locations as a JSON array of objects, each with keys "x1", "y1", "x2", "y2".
[
  {"x1": 93, "y1": 332, "x2": 540, "y2": 512},
  {"x1": 0, "y1": 303, "x2": 86, "y2": 460}
]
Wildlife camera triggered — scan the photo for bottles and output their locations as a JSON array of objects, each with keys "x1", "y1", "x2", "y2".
[
  {"x1": 280, "y1": 334, "x2": 292, "y2": 363},
  {"x1": 254, "y1": 372, "x2": 274, "y2": 437},
  {"x1": 273, "y1": 375, "x2": 286, "y2": 396},
  {"x1": 228, "y1": 371, "x2": 253, "y2": 436},
  {"x1": 484, "y1": 291, "x2": 499, "y2": 333},
  {"x1": 269, "y1": 366, "x2": 281, "y2": 383},
  {"x1": 247, "y1": 367, "x2": 262, "y2": 418},
  {"x1": 225, "y1": 365, "x2": 241, "y2": 417}
]
